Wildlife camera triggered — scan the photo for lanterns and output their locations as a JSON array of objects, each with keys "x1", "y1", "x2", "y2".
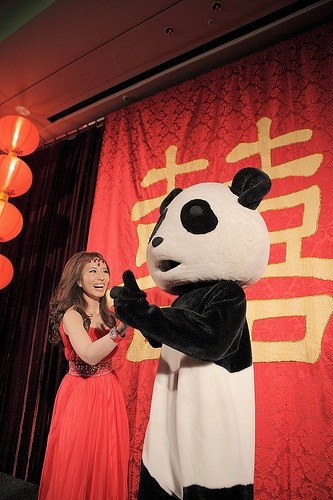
[{"x1": 0, "y1": 115, "x2": 38, "y2": 291}]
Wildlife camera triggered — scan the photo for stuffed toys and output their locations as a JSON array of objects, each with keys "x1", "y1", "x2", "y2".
[{"x1": 110, "y1": 167, "x2": 269, "y2": 500}]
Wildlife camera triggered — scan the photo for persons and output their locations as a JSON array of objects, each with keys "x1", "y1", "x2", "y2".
[{"x1": 36, "y1": 251, "x2": 131, "y2": 500}]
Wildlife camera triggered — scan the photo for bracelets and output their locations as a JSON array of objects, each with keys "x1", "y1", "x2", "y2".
[{"x1": 111, "y1": 326, "x2": 125, "y2": 343}]
